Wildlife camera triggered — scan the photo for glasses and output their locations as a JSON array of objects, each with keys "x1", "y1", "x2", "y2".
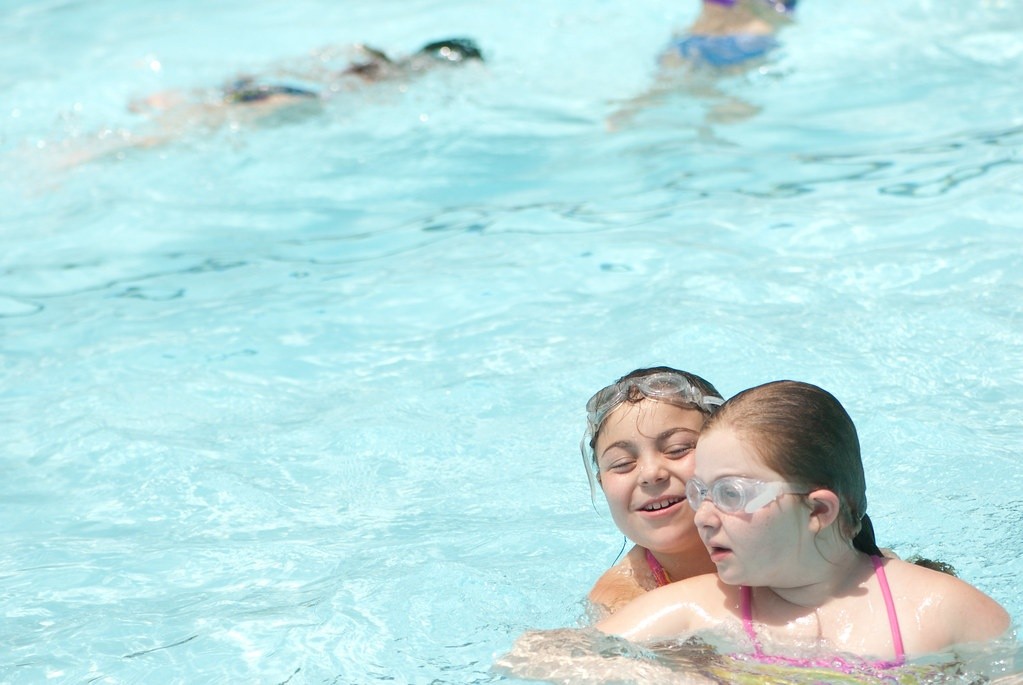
[
  {"x1": 684, "y1": 475, "x2": 811, "y2": 515},
  {"x1": 586, "y1": 372, "x2": 725, "y2": 439}
]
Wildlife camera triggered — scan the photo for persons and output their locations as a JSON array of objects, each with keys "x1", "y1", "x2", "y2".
[
  {"x1": 594, "y1": 380, "x2": 1012, "y2": 661},
  {"x1": 587, "y1": 367, "x2": 727, "y2": 611}
]
